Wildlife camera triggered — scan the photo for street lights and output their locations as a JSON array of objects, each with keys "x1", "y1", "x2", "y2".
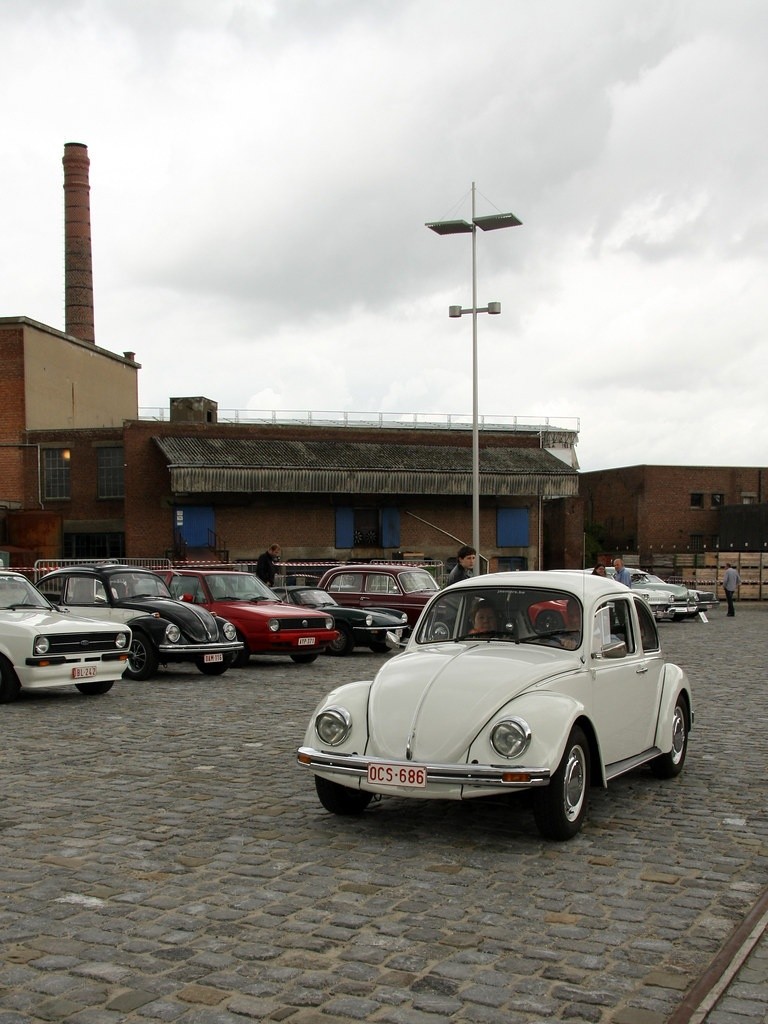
[{"x1": 448, "y1": 302, "x2": 501, "y2": 577}]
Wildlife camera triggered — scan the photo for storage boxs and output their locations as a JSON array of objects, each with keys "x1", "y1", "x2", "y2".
[{"x1": 591, "y1": 552, "x2": 768, "y2": 602}]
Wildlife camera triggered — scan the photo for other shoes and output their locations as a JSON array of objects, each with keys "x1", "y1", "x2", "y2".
[{"x1": 727, "y1": 613, "x2": 734, "y2": 616}]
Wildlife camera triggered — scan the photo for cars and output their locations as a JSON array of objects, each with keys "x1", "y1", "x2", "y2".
[
  {"x1": 112, "y1": 570, "x2": 339, "y2": 668},
  {"x1": 296, "y1": 571, "x2": 695, "y2": 842},
  {"x1": 646, "y1": 575, "x2": 720, "y2": 619},
  {"x1": 551, "y1": 570, "x2": 676, "y2": 626},
  {"x1": 270, "y1": 585, "x2": 412, "y2": 657},
  {"x1": 588, "y1": 567, "x2": 699, "y2": 622},
  {"x1": 21, "y1": 563, "x2": 244, "y2": 681},
  {"x1": 318, "y1": 565, "x2": 484, "y2": 639},
  {"x1": 528, "y1": 600, "x2": 581, "y2": 636},
  {"x1": 0, "y1": 571, "x2": 133, "y2": 704}
]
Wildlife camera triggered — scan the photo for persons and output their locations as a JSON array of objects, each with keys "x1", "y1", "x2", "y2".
[
  {"x1": 255, "y1": 544, "x2": 283, "y2": 586},
  {"x1": 549, "y1": 599, "x2": 627, "y2": 658},
  {"x1": 591, "y1": 563, "x2": 606, "y2": 577},
  {"x1": 722, "y1": 563, "x2": 740, "y2": 617},
  {"x1": 446, "y1": 545, "x2": 476, "y2": 586},
  {"x1": 613, "y1": 558, "x2": 631, "y2": 589},
  {"x1": 468, "y1": 600, "x2": 497, "y2": 635}
]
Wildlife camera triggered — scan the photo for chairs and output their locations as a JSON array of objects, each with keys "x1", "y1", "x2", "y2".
[
  {"x1": 110, "y1": 582, "x2": 126, "y2": 599},
  {"x1": 70, "y1": 580, "x2": 95, "y2": 603}
]
